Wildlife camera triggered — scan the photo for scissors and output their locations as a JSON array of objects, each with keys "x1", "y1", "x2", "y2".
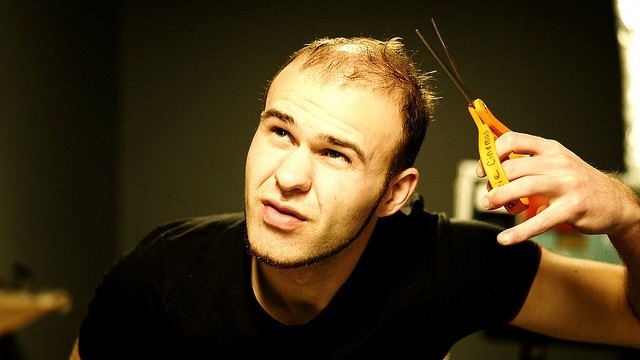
[{"x1": 416, "y1": 18, "x2": 530, "y2": 215}]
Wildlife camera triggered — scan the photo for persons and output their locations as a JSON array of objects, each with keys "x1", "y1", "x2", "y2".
[{"x1": 66, "y1": 34, "x2": 639, "y2": 359}]
